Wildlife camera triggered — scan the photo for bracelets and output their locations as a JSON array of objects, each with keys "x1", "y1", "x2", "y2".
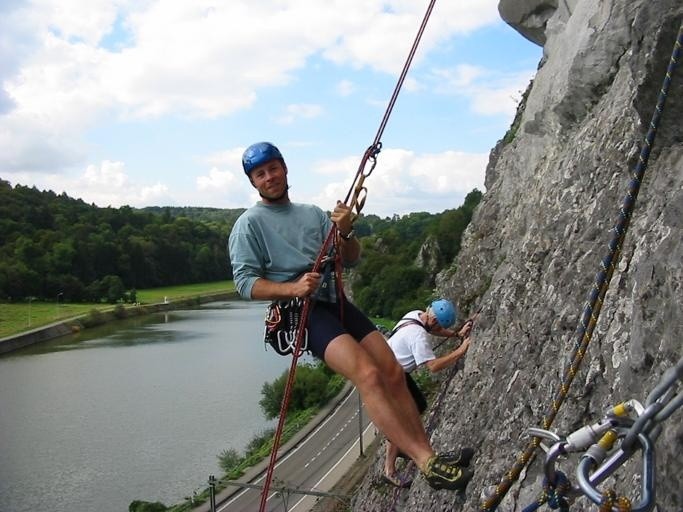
[{"x1": 456, "y1": 332, "x2": 458, "y2": 337}]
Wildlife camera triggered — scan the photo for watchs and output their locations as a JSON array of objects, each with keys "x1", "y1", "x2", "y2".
[{"x1": 338, "y1": 225, "x2": 355, "y2": 240}]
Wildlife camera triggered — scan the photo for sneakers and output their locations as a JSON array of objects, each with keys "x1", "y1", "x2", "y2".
[
  {"x1": 423, "y1": 447, "x2": 473, "y2": 490},
  {"x1": 384, "y1": 473, "x2": 413, "y2": 489}
]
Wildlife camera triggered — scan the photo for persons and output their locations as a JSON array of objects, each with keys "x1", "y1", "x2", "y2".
[
  {"x1": 228, "y1": 142, "x2": 474, "y2": 490},
  {"x1": 378, "y1": 297, "x2": 470, "y2": 487}
]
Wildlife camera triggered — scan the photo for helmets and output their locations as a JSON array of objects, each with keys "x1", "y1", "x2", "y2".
[
  {"x1": 431, "y1": 300, "x2": 457, "y2": 330},
  {"x1": 242, "y1": 143, "x2": 282, "y2": 175}
]
